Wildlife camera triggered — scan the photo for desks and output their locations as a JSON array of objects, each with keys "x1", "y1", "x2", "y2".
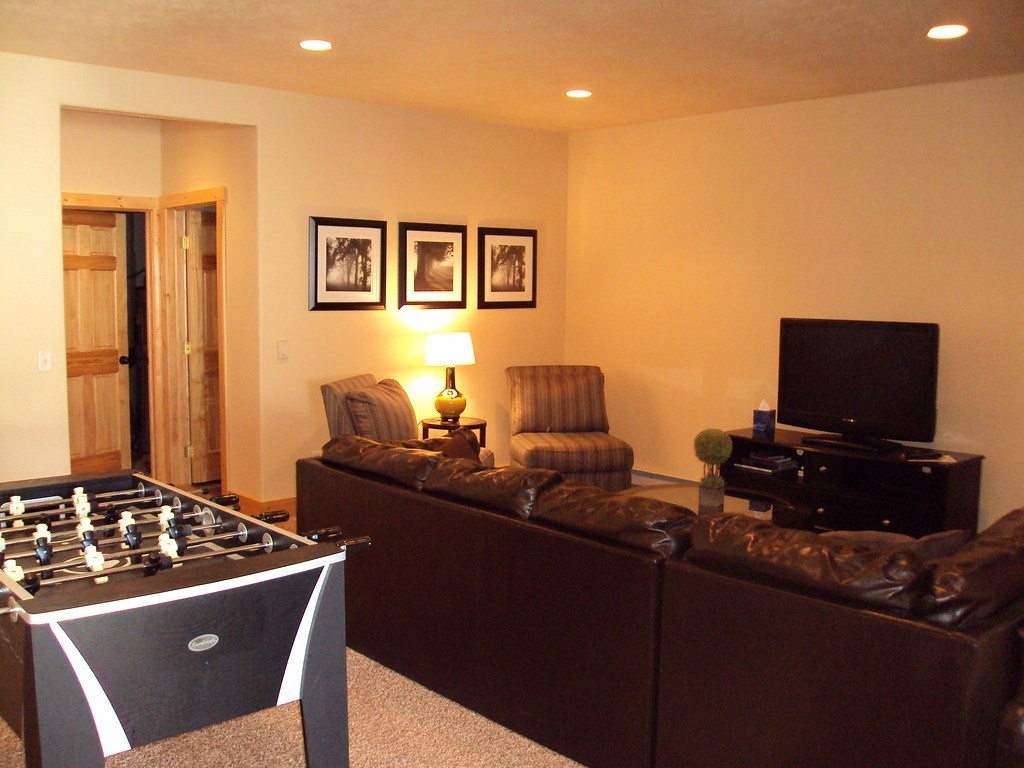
[
  {"x1": 717, "y1": 423, "x2": 984, "y2": 541},
  {"x1": 421, "y1": 414, "x2": 487, "y2": 448}
]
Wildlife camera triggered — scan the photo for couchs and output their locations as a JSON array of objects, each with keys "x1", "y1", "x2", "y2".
[{"x1": 296, "y1": 432, "x2": 1023, "y2": 768}]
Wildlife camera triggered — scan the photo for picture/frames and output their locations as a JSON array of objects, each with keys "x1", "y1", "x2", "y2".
[
  {"x1": 308, "y1": 216, "x2": 387, "y2": 310},
  {"x1": 477, "y1": 226, "x2": 537, "y2": 309},
  {"x1": 396, "y1": 222, "x2": 469, "y2": 310}
]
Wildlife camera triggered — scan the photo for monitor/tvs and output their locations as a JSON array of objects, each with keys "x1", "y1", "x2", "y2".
[{"x1": 776, "y1": 317, "x2": 939, "y2": 443}]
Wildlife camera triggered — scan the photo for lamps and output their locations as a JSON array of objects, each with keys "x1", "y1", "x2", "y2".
[{"x1": 424, "y1": 331, "x2": 477, "y2": 422}]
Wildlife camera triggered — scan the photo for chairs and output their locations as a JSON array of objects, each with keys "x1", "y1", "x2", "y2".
[
  {"x1": 504, "y1": 365, "x2": 634, "y2": 494},
  {"x1": 320, "y1": 372, "x2": 419, "y2": 447}
]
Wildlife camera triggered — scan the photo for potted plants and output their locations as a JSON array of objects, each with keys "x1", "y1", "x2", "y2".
[{"x1": 693, "y1": 428, "x2": 732, "y2": 519}]
